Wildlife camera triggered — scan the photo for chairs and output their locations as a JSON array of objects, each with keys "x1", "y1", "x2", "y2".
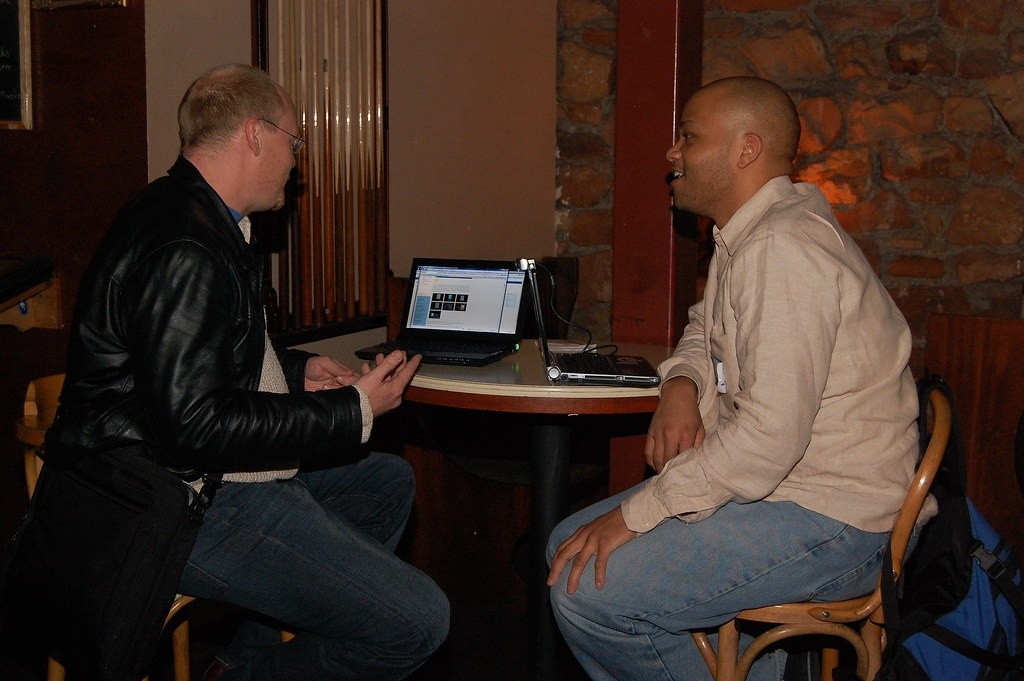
[
  {"x1": 0, "y1": 272, "x2": 295, "y2": 681},
  {"x1": 691, "y1": 378, "x2": 948, "y2": 681}
]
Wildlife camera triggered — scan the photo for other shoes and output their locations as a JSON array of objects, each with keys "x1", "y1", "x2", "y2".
[{"x1": 202, "y1": 657, "x2": 232, "y2": 681}]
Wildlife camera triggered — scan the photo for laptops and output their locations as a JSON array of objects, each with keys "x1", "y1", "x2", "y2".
[
  {"x1": 529, "y1": 259, "x2": 661, "y2": 388},
  {"x1": 355, "y1": 257, "x2": 532, "y2": 368}
]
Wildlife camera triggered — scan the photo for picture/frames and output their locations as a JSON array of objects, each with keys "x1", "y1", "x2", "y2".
[{"x1": 0, "y1": 0, "x2": 34, "y2": 132}]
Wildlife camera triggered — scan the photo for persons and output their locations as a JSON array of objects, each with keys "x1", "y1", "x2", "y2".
[
  {"x1": 25, "y1": 63, "x2": 449, "y2": 681},
  {"x1": 547, "y1": 76, "x2": 937, "y2": 680}
]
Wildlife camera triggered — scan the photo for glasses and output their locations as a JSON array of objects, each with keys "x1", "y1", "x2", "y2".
[{"x1": 244, "y1": 118, "x2": 306, "y2": 154}]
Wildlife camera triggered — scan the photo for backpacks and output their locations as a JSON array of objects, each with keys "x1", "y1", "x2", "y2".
[{"x1": 873, "y1": 369, "x2": 1024, "y2": 681}]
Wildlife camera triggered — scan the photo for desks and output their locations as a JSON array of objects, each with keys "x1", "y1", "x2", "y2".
[{"x1": 285, "y1": 326, "x2": 675, "y2": 681}]
